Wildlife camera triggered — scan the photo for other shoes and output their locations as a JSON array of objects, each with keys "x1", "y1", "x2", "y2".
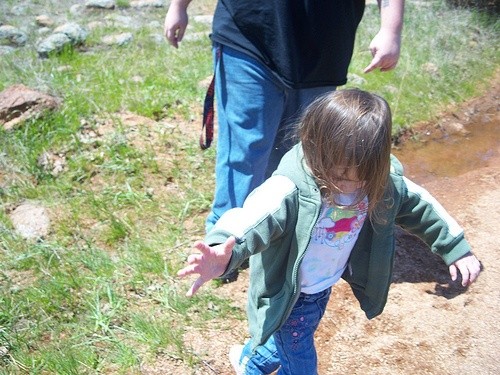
[{"x1": 222, "y1": 259, "x2": 250, "y2": 284}]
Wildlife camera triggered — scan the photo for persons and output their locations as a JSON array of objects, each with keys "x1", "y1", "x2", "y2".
[
  {"x1": 163, "y1": 0, "x2": 405, "y2": 284},
  {"x1": 177, "y1": 87, "x2": 480, "y2": 375}
]
